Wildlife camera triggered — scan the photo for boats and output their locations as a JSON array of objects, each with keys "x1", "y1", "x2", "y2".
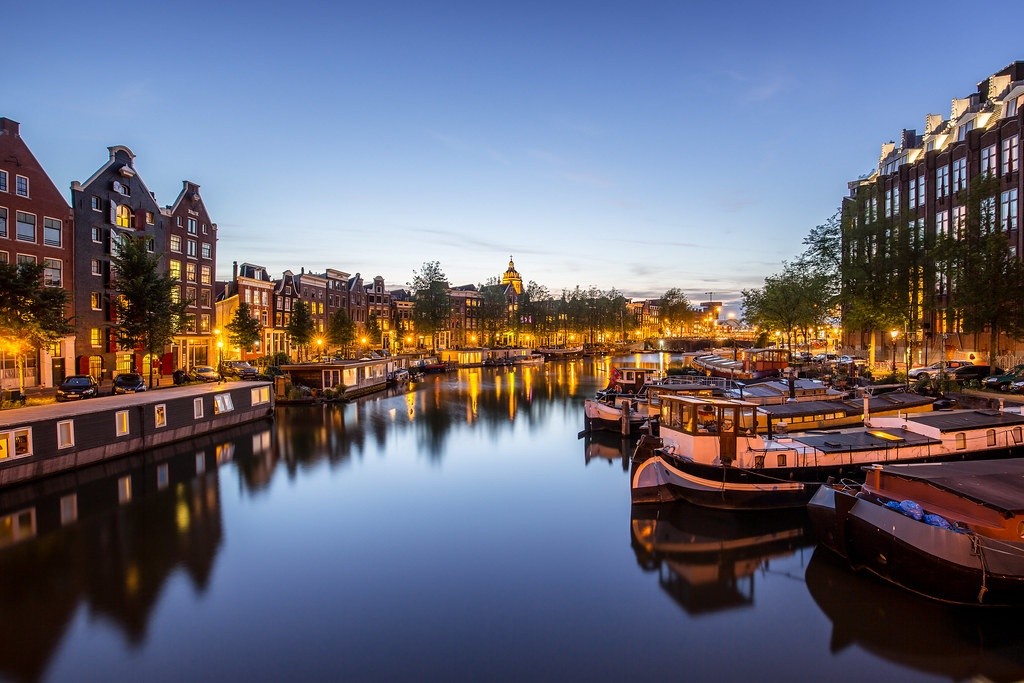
[
  {"x1": 481, "y1": 360, "x2": 512, "y2": 366},
  {"x1": 0, "y1": 380, "x2": 276, "y2": 488},
  {"x1": 630, "y1": 396, "x2": 1024, "y2": 513},
  {"x1": 540, "y1": 346, "x2": 583, "y2": 359},
  {"x1": 279, "y1": 351, "x2": 441, "y2": 407},
  {"x1": 513, "y1": 353, "x2": 545, "y2": 364},
  {"x1": 577, "y1": 367, "x2": 844, "y2": 442},
  {"x1": 805, "y1": 460, "x2": 1024, "y2": 609},
  {"x1": 690, "y1": 348, "x2": 790, "y2": 381},
  {"x1": 643, "y1": 382, "x2": 934, "y2": 435},
  {"x1": 440, "y1": 346, "x2": 532, "y2": 368}
]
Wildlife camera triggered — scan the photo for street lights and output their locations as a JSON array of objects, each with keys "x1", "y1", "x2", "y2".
[
  {"x1": 215, "y1": 329, "x2": 223, "y2": 360},
  {"x1": 217, "y1": 342, "x2": 223, "y2": 369},
  {"x1": 891, "y1": 329, "x2": 898, "y2": 372}
]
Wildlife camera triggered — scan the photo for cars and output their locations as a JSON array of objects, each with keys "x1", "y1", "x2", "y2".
[
  {"x1": 947, "y1": 364, "x2": 1006, "y2": 389},
  {"x1": 313, "y1": 355, "x2": 333, "y2": 362},
  {"x1": 372, "y1": 350, "x2": 391, "y2": 357},
  {"x1": 219, "y1": 360, "x2": 259, "y2": 381},
  {"x1": 1010, "y1": 382, "x2": 1024, "y2": 394},
  {"x1": 55, "y1": 374, "x2": 98, "y2": 402},
  {"x1": 791, "y1": 351, "x2": 868, "y2": 368},
  {"x1": 367, "y1": 351, "x2": 380, "y2": 358},
  {"x1": 188, "y1": 366, "x2": 222, "y2": 383},
  {"x1": 112, "y1": 372, "x2": 146, "y2": 395},
  {"x1": 982, "y1": 364, "x2": 1024, "y2": 392},
  {"x1": 908, "y1": 361, "x2": 974, "y2": 383}
]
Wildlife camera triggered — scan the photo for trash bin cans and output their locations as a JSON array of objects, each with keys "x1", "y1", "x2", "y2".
[{"x1": 4, "y1": 390, "x2": 20, "y2": 404}]
